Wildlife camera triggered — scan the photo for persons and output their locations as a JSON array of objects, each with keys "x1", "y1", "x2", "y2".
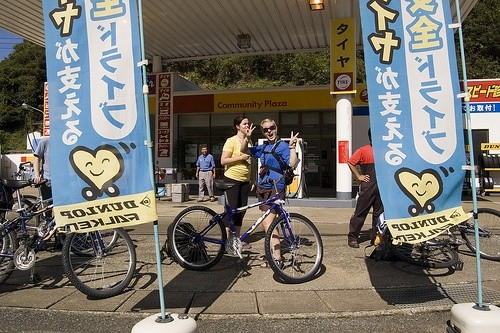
[
  {"x1": 347, "y1": 127, "x2": 386, "y2": 248},
  {"x1": 220, "y1": 115, "x2": 251, "y2": 251},
  {"x1": 33, "y1": 136, "x2": 54, "y2": 218},
  {"x1": 196, "y1": 144, "x2": 217, "y2": 202},
  {"x1": 240, "y1": 118, "x2": 299, "y2": 269}
]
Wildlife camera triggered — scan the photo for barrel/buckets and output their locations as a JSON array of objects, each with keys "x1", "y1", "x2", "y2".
[{"x1": 157, "y1": 187, "x2": 166, "y2": 196}]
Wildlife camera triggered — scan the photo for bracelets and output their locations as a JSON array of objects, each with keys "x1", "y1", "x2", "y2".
[{"x1": 288, "y1": 146, "x2": 296, "y2": 149}]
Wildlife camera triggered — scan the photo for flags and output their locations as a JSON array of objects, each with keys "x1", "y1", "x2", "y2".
[
  {"x1": 359, "y1": 0, "x2": 471, "y2": 247},
  {"x1": 42, "y1": 0, "x2": 159, "y2": 234}
]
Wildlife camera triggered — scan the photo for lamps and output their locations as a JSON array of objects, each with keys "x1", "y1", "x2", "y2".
[
  {"x1": 309, "y1": 0, "x2": 324, "y2": 10},
  {"x1": 237, "y1": 34, "x2": 251, "y2": 49}
]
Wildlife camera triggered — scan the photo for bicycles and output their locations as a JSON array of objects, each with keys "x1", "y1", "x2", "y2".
[
  {"x1": 391, "y1": 207, "x2": 500, "y2": 270},
  {"x1": 0, "y1": 161, "x2": 120, "y2": 257},
  {"x1": 169, "y1": 169, "x2": 324, "y2": 284},
  {"x1": 0, "y1": 179, "x2": 136, "y2": 300}
]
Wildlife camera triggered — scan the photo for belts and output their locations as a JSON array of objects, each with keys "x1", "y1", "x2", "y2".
[{"x1": 200, "y1": 170, "x2": 210, "y2": 172}]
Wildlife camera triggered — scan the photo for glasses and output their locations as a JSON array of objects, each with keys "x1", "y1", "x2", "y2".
[{"x1": 262, "y1": 126, "x2": 275, "y2": 132}]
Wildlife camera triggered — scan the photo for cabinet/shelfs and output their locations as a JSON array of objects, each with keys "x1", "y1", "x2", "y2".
[{"x1": 481, "y1": 143, "x2": 500, "y2": 196}]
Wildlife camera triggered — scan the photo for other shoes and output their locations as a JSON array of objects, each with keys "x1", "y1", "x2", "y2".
[
  {"x1": 197, "y1": 199, "x2": 204, "y2": 202},
  {"x1": 370, "y1": 238, "x2": 376, "y2": 244},
  {"x1": 349, "y1": 241, "x2": 360, "y2": 248},
  {"x1": 210, "y1": 196, "x2": 217, "y2": 201},
  {"x1": 240, "y1": 241, "x2": 251, "y2": 249},
  {"x1": 258, "y1": 255, "x2": 266, "y2": 261},
  {"x1": 261, "y1": 261, "x2": 283, "y2": 269}
]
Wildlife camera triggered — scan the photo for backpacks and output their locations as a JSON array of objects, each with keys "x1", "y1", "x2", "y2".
[
  {"x1": 167, "y1": 222, "x2": 210, "y2": 265},
  {"x1": 260, "y1": 142, "x2": 294, "y2": 184}
]
